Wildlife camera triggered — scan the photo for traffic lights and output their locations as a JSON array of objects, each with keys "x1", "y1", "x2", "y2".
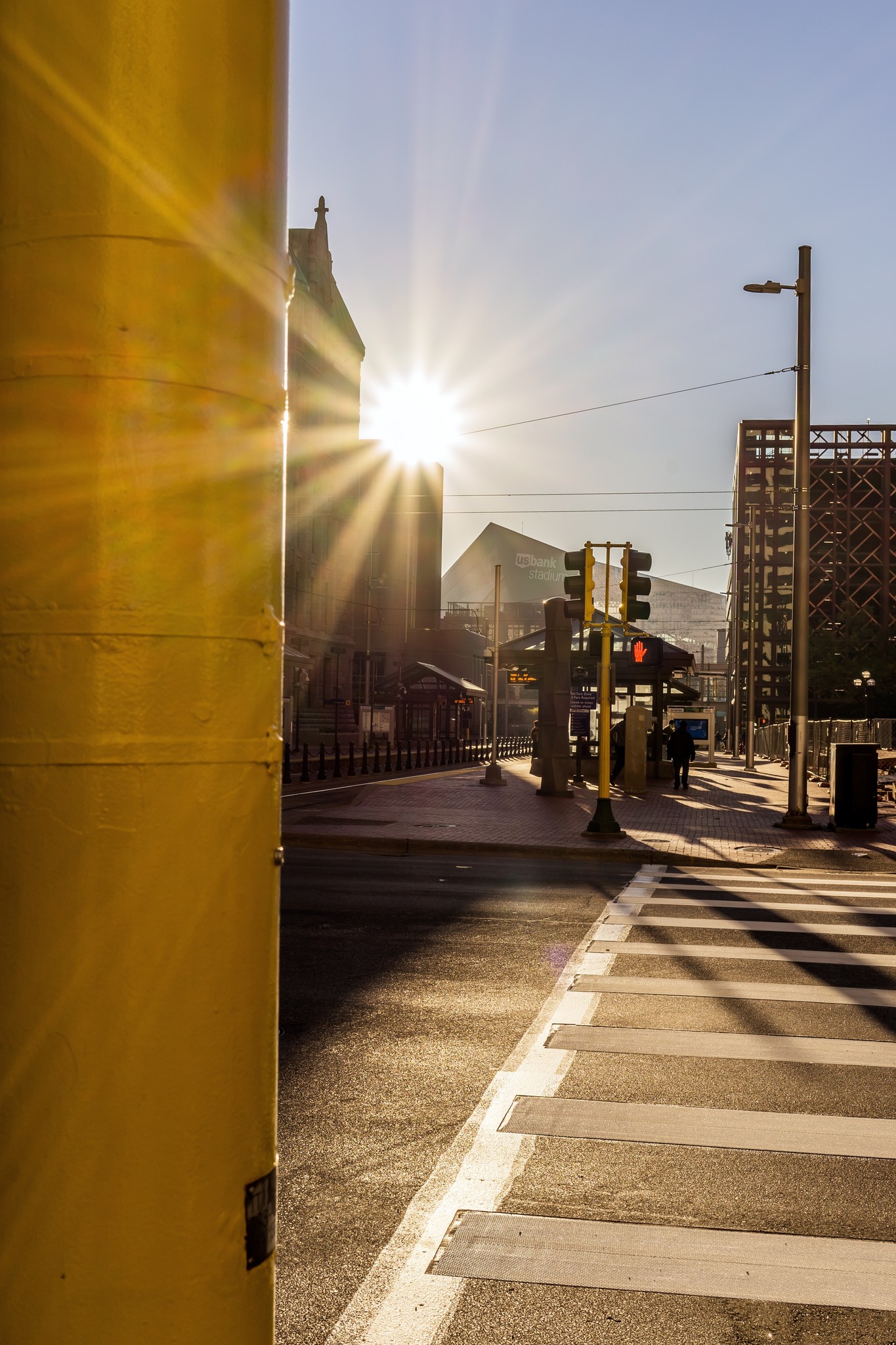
[
  {"x1": 629, "y1": 636, "x2": 665, "y2": 667},
  {"x1": 762, "y1": 718, "x2": 768, "y2": 724},
  {"x1": 562, "y1": 542, "x2": 596, "y2": 623},
  {"x1": 625, "y1": 548, "x2": 655, "y2": 625}
]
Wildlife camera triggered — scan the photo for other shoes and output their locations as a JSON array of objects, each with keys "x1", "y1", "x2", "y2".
[
  {"x1": 674, "y1": 786, "x2": 678, "y2": 788},
  {"x1": 610, "y1": 776, "x2": 615, "y2": 785},
  {"x1": 683, "y1": 786, "x2": 688, "y2": 789}
]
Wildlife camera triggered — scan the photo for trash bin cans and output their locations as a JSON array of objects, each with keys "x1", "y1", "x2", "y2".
[{"x1": 828, "y1": 743, "x2": 881, "y2": 832}]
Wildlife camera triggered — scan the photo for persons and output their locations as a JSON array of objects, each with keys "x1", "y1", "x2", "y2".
[
  {"x1": 531, "y1": 720, "x2": 538, "y2": 765},
  {"x1": 667, "y1": 720, "x2": 696, "y2": 789},
  {"x1": 609, "y1": 712, "x2": 627, "y2": 786},
  {"x1": 714, "y1": 726, "x2": 757, "y2": 757},
  {"x1": 663, "y1": 719, "x2": 676, "y2": 745}
]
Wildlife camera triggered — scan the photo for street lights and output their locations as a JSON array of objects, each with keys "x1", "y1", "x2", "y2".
[
  {"x1": 740, "y1": 246, "x2": 814, "y2": 829},
  {"x1": 854, "y1": 670, "x2": 875, "y2": 742}
]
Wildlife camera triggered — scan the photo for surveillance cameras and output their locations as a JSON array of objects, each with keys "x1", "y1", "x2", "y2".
[
  {"x1": 403, "y1": 690, "x2": 407, "y2": 695},
  {"x1": 305, "y1": 677, "x2": 310, "y2": 683},
  {"x1": 459, "y1": 694, "x2": 464, "y2": 699},
  {"x1": 482, "y1": 648, "x2": 492, "y2": 662}
]
